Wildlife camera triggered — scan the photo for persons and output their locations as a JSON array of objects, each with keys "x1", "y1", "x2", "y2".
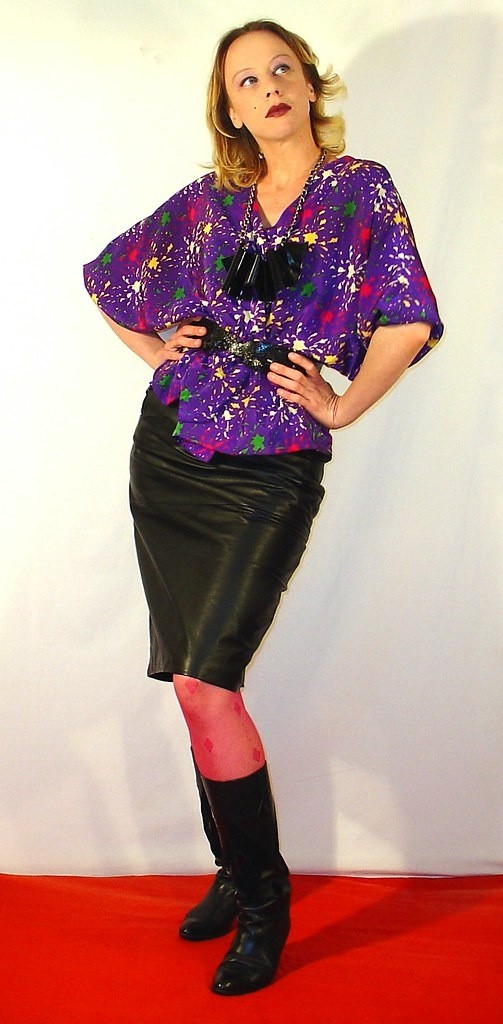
[{"x1": 81, "y1": 18, "x2": 443, "y2": 995}]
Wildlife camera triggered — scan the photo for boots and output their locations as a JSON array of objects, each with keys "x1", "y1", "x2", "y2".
[
  {"x1": 180, "y1": 746, "x2": 241, "y2": 940},
  {"x1": 200, "y1": 759, "x2": 291, "y2": 995}
]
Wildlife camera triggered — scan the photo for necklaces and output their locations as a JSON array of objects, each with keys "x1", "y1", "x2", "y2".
[{"x1": 222, "y1": 146, "x2": 328, "y2": 304}]
